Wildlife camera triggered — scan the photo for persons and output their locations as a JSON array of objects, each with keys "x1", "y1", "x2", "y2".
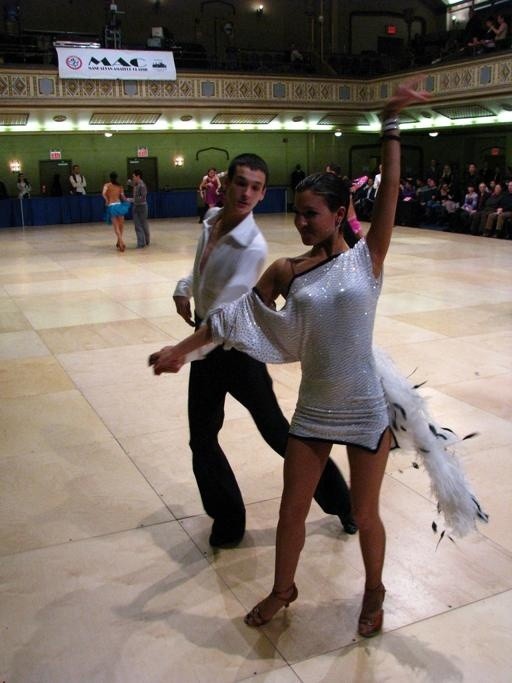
[
  {"x1": 283, "y1": 7, "x2": 512, "y2": 81},
  {"x1": 149, "y1": 154, "x2": 372, "y2": 547},
  {"x1": 290, "y1": 164, "x2": 305, "y2": 199},
  {"x1": 399, "y1": 161, "x2": 511, "y2": 238},
  {"x1": 199, "y1": 167, "x2": 229, "y2": 223},
  {"x1": 154, "y1": 67, "x2": 435, "y2": 639},
  {"x1": 1, "y1": 163, "x2": 163, "y2": 257},
  {"x1": 352, "y1": 163, "x2": 383, "y2": 220}
]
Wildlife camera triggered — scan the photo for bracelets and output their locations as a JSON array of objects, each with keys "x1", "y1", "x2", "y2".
[{"x1": 376, "y1": 104, "x2": 405, "y2": 143}]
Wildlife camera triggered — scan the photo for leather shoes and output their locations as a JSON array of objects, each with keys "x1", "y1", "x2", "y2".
[{"x1": 337, "y1": 487, "x2": 359, "y2": 535}]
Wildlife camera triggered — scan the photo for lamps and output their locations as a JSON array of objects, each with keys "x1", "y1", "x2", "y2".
[
  {"x1": 174, "y1": 156, "x2": 184, "y2": 165},
  {"x1": 256, "y1": 4, "x2": 264, "y2": 16},
  {"x1": 11, "y1": 160, "x2": 20, "y2": 172}
]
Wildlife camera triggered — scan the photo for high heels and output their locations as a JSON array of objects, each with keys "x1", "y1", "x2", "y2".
[
  {"x1": 357, "y1": 582, "x2": 386, "y2": 636},
  {"x1": 244, "y1": 582, "x2": 299, "y2": 628}
]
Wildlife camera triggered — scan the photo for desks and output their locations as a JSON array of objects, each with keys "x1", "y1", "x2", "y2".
[{"x1": 0, "y1": 185, "x2": 288, "y2": 225}]
